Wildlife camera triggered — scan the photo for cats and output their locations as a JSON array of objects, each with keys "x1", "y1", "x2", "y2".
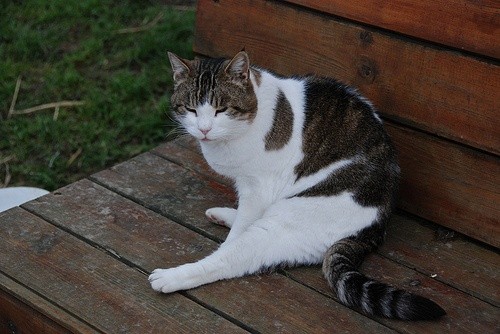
[{"x1": 149, "y1": 48, "x2": 448, "y2": 322}]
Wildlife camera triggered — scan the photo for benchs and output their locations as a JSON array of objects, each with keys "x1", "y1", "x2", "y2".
[{"x1": 0, "y1": 0, "x2": 500, "y2": 333}]
